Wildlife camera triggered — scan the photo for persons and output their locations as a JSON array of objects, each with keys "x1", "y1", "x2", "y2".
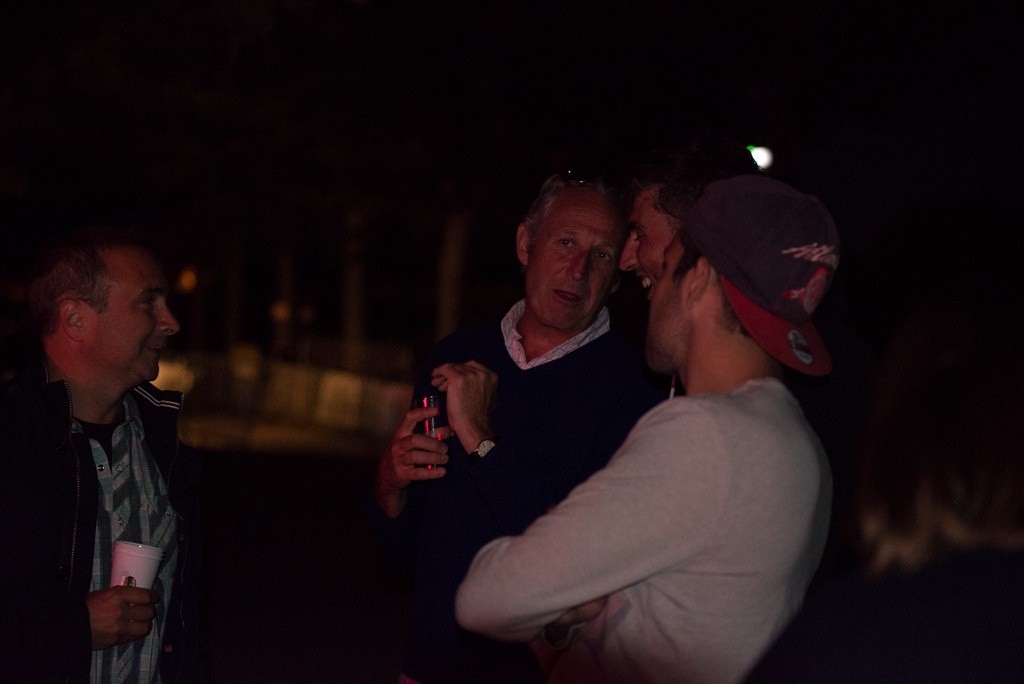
[
  {"x1": 371, "y1": 141, "x2": 839, "y2": 683},
  {"x1": 0, "y1": 224, "x2": 200, "y2": 684}
]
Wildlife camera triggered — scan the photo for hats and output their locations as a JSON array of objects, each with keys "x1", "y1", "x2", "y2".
[{"x1": 681, "y1": 173, "x2": 840, "y2": 377}]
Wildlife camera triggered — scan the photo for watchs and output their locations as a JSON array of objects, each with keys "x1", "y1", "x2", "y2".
[{"x1": 467, "y1": 440, "x2": 499, "y2": 460}]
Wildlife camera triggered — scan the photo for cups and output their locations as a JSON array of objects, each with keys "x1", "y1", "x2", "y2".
[
  {"x1": 109, "y1": 540, "x2": 165, "y2": 623},
  {"x1": 411, "y1": 396, "x2": 453, "y2": 469}
]
